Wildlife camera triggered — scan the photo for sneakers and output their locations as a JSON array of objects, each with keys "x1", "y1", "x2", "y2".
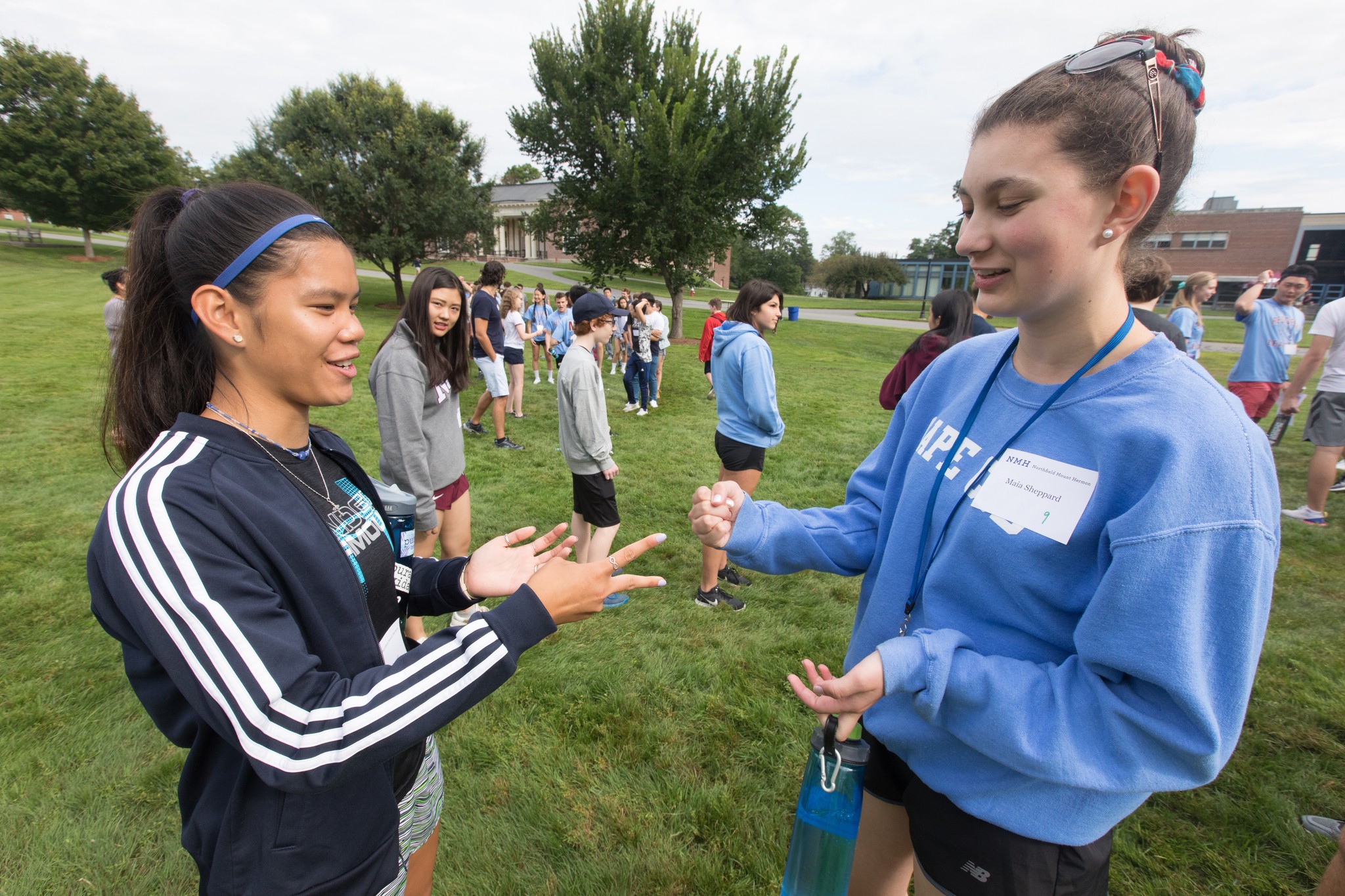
[
  {"x1": 716, "y1": 564, "x2": 753, "y2": 588},
  {"x1": 612, "y1": 566, "x2": 624, "y2": 576},
  {"x1": 1281, "y1": 504, "x2": 1328, "y2": 527},
  {"x1": 649, "y1": 400, "x2": 658, "y2": 408},
  {"x1": 623, "y1": 403, "x2": 640, "y2": 412},
  {"x1": 601, "y1": 593, "x2": 630, "y2": 608},
  {"x1": 694, "y1": 583, "x2": 747, "y2": 613},
  {"x1": 636, "y1": 408, "x2": 648, "y2": 416},
  {"x1": 1331, "y1": 477, "x2": 1345, "y2": 492},
  {"x1": 463, "y1": 418, "x2": 489, "y2": 435},
  {"x1": 620, "y1": 368, "x2": 626, "y2": 374},
  {"x1": 610, "y1": 370, "x2": 616, "y2": 374},
  {"x1": 625, "y1": 401, "x2": 639, "y2": 406},
  {"x1": 494, "y1": 438, "x2": 525, "y2": 451}
]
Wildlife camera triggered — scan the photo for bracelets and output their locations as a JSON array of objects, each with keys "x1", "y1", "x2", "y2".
[{"x1": 460, "y1": 561, "x2": 482, "y2": 600}]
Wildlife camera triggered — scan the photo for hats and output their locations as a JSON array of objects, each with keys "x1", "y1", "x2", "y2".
[{"x1": 572, "y1": 292, "x2": 630, "y2": 325}]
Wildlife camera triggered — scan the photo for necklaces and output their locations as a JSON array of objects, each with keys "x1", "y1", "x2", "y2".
[
  {"x1": 206, "y1": 406, "x2": 345, "y2": 517},
  {"x1": 206, "y1": 401, "x2": 312, "y2": 460}
]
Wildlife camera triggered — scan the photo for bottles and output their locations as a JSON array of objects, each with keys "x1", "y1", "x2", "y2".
[
  {"x1": 780, "y1": 714, "x2": 870, "y2": 896},
  {"x1": 620, "y1": 341, "x2": 625, "y2": 351},
  {"x1": 374, "y1": 484, "x2": 417, "y2": 633},
  {"x1": 548, "y1": 348, "x2": 559, "y2": 359},
  {"x1": 1267, "y1": 412, "x2": 1291, "y2": 446}
]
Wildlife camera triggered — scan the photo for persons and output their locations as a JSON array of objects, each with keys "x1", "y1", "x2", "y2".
[
  {"x1": 100, "y1": 267, "x2": 129, "y2": 356},
  {"x1": 689, "y1": 24, "x2": 1280, "y2": 896},
  {"x1": 85, "y1": 181, "x2": 667, "y2": 895},
  {"x1": 458, "y1": 254, "x2": 1345, "y2": 610},
  {"x1": 368, "y1": 267, "x2": 491, "y2": 644}
]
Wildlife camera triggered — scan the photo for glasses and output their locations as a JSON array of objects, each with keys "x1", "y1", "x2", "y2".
[
  {"x1": 597, "y1": 318, "x2": 616, "y2": 327},
  {"x1": 1064, "y1": 29, "x2": 1163, "y2": 175}
]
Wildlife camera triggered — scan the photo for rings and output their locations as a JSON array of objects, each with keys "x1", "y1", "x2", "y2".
[
  {"x1": 608, "y1": 556, "x2": 618, "y2": 571},
  {"x1": 532, "y1": 541, "x2": 539, "y2": 553},
  {"x1": 504, "y1": 534, "x2": 512, "y2": 546},
  {"x1": 534, "y1": 565, "x2": 537, "y2": 572}
]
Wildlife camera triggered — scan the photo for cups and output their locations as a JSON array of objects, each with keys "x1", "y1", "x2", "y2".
[{"x1": 537, "y1": 325, "x2": 544, "y2": 331}]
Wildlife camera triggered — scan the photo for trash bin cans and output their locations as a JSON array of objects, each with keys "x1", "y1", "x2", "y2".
[{"x1": 788, "y1": 306, "x2": 799, "y2": 321}]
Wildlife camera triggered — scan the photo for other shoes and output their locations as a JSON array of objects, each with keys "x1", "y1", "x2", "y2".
[
  {"x1": 656, "y1": 389, "x2": 662, "y2": 399},
  {"x1": 510, "y1": 411, "x2": 528, "y2": 418},
  {"x1": 547, "y1": 378, "x2": 555, "y2": 384},
  {"x1": 475, "y1": 372, "x2": 484, "y2": 381},
  {"x1": 606, "y1": 357, "x2": 612, "y2": 360},
  {"x1": 707, "y1": 388, "x2": 714, "y2": 400},
  {"x1": 533, "y1": 379, "x2": 541, "y2": 384}
]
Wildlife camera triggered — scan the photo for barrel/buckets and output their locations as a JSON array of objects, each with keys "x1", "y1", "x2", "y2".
[{"x1": 788, "y1": 306, "x2": 799, "y2": 321}]
